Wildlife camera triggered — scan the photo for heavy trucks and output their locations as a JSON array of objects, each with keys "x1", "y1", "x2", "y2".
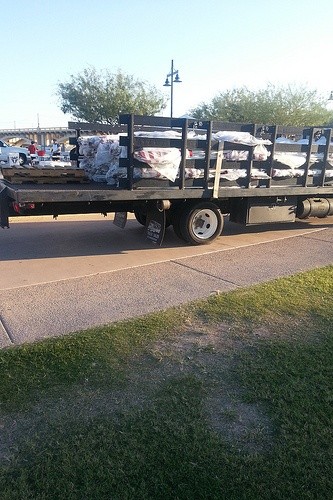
[{"x1": 1, "y1": 114, "x2": 333, "y2": 245}]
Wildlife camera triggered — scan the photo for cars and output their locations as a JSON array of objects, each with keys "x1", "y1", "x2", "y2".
[{"x1": 0, "y1": 139, "x2": 32, "y2": 165}]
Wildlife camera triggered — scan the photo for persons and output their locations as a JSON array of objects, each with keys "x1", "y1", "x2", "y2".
[{"x1": 28, "y1": 139, "x2": 62, "y2": 160}]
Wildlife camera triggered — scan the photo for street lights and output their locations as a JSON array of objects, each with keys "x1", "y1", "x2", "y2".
[{"x1": 162, "y1": 59, "x2": 183, "y2": 130}]
